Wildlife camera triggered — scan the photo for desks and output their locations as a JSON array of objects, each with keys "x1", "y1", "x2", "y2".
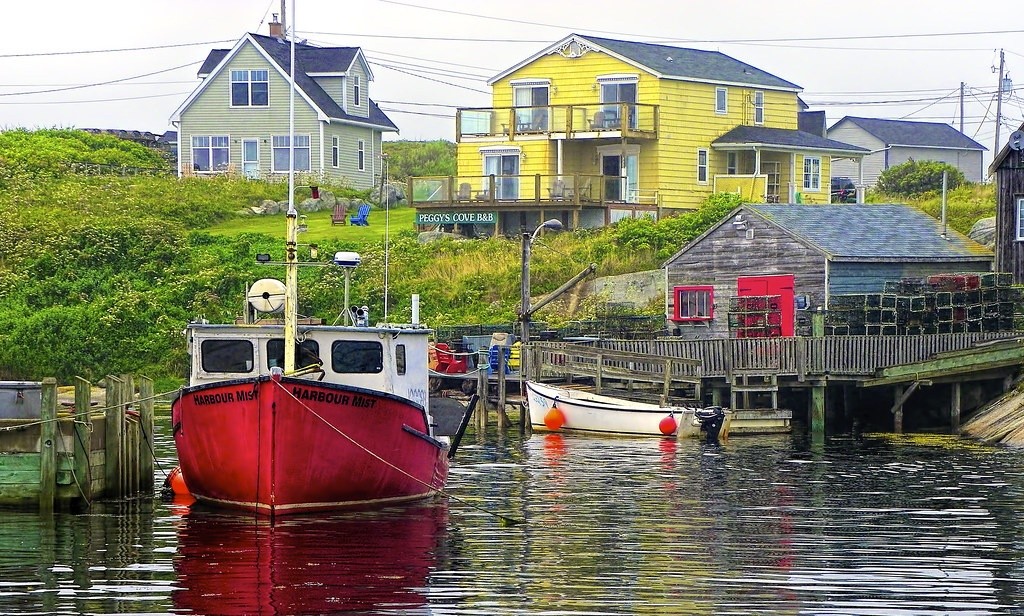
[
  {"x1": 501, "y1": 123, "x2": 531, "y2": 136},
  {"x1": 604, "y1": 118, "x2": 615, "y2": 128},
  {"x1": 454, "y1": 190, "x2": 498, "y2": 202},
  {"x1": 547, "y1": 187, "x2": 589, "y2": 201}
]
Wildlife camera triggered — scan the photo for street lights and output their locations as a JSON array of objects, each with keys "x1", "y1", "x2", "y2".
[{"x1": 518, "y1": 218, "x2": 563, "y2": 378}]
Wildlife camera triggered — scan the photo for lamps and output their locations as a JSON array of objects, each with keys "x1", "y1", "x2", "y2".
[{"x1": 553, "y1": 87, "x2": 558, "y2": 95}]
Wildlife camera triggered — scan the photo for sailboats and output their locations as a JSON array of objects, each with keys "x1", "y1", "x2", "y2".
[{"x1": 169, "y1": 0, "x2": 451, "y2": 517}]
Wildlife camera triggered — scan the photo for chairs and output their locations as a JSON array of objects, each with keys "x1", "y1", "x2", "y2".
[
  {"x1": 587, "y1": 112, "x2": 605, "y2": 132},
  {"x1": 614, "y1": 110, "x2": 633, "y2": 127},
  {"x1": 350, "y1": 203, "x2": 371, "y2": 226},
  {"x1": 428, "y1": 340, "x2": 519, "y2": 374},
  {"x1": 454, "y1": 182, "x2": 472, "y2": 202},
  {"x1": 475, "y1": 182, "x2": 500, "y2": 202},
  {"x1": 330, "y1": 203, "x2": 348, "y2": 226},
  {"x1": 568, "y1": 177, "x2": 592, "y2": 202},
  {"x1": 546, "y1": 180, "x2": 564, "y2": 201}
]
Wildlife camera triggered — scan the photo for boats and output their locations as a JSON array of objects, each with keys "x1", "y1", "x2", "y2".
[{"x1": 525, "y1": 379, "x2": 734, "y2": 438}]
